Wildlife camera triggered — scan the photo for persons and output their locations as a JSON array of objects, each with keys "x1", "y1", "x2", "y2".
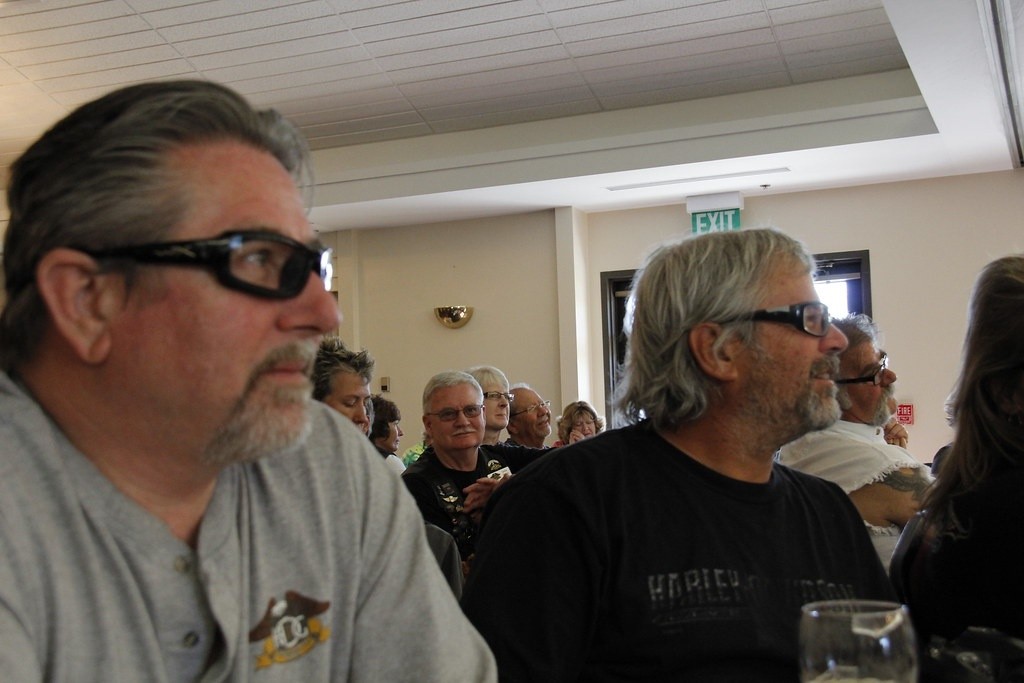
[
  {"x1": 466, "y1": 366, "x2": 514, "y2": 445},
  {"x1": 552, "y1": 401, "x2": 602, "y2": 447},
  {"x1": 902, "y1": 257, "x2": 1024, "y2": 645},
  {"x1": 0, "y1": 79, "x2": 496, "y2": 683},
  {"x1": 368, "y1": 395, "x2": 406, "y2": 475},
  {"x1": 400, "y1": 370, "x2": 557, "y2": 578},
  {"x1": 505, "y1": 386, "x2": 552, "y2": 449},
  {"x1": 778, "y1": 314, "x2": 934, "y2": 575},
  {"x1": 310, "y1": 336, "x2": 374, "y2": 438},
  {"x1": 457, "y1": 228, "x2": 900, "y2": 683}
]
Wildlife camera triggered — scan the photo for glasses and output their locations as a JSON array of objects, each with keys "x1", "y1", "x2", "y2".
[
  {"x1": 483, "y1": 392, "x2": 514, "y2": 402},
  {"x1": 428, "y1": 405, "x2": 483, "y2": 421},
  {"x1": 836, "y1": 355, "x2": 888, "y2": 386},
  {"x1": 719, "y1": 301, "x2": 829, "y2": 336},
  {"x1": 11, "y1": 227, "x2": 332, "y2": 300},
  {"x1": 510, "y1": 401, "x2": 550, "y2": 417}
]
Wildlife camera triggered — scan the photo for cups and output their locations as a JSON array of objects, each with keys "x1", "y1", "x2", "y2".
[{"x1": 798, "y1": 598, "x2": 919, "y2": 683}]
